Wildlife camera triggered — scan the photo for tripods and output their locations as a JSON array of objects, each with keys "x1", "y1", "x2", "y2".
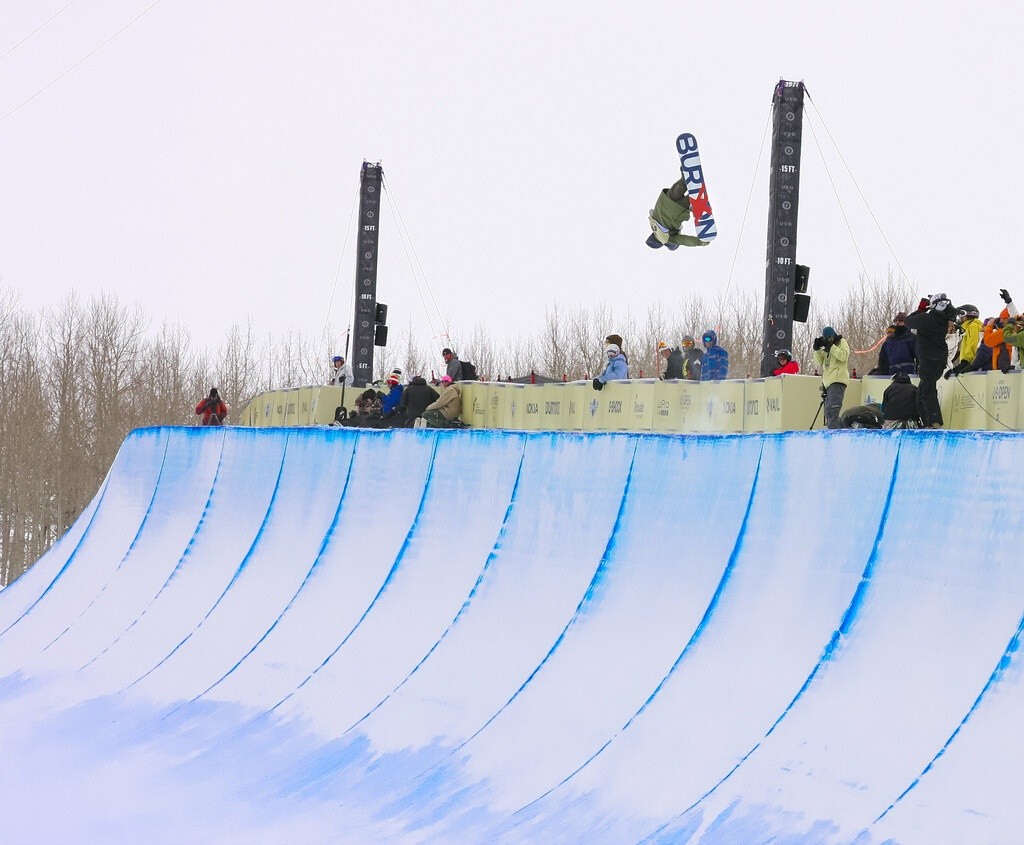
[{"x1": 810, "y1": 393, "x2": 827, "y2": 430}]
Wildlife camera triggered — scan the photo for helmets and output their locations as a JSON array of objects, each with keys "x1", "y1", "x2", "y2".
[
  {"x1": 332, "y1": 356, "x2": 344, "y2": 367},
  {"x1": 777, "y1": 349, "x2": 791, "y2": 366},
  {"x1": 896, "y1": 312, "x2": 908, "y2": 319},
  {"x1": 957, "y1": 305, "x2": 979, "y2": 319}
]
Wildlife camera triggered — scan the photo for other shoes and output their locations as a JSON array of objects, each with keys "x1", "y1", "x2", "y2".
[{"x1": 852, "y1": 419, "x2": 941, "y2": 430}]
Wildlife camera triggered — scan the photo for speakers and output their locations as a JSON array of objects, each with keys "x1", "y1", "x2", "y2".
[
  {"x1": 795, "y1": 264, "x2": 810, "y2": 293},
  {"x1": 375, "y1": 326, "x2": 388, "y2": 346},
  {"x1": 793, "y1": 294, "x2": 811, "y2": 323},
  {"x1": 375, "y1": 304, "x2": 388, "y2": 325}
]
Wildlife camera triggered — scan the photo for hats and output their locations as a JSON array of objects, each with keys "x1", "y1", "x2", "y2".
[
  {"x1": 442, "y1": 348, "x2": 451, "y2": 356},
  {"x1": 210, "y1": 389, "x2": 219, "y2": 397},
  {"x1": 645, "y1": 233, "x2": 663, "y2": 249},
  {"x1": 822, "y1": 327, "x2": 836, "y2": 338},
  {"x1": 607, "y1": 343, "x2": 620, "y2": 357},
  {"x1": 356, "y1": 368, "x2": 452, "y2": 406},
  {"x1": 999, "y1": 308, "x2": 1009, "y2": 319},
  {"x1": 983, "y1": 317, "x2": 997, "y2": 326},
  {"x1": 930, "y1": 293, "x2": 947, "y2": 311}
]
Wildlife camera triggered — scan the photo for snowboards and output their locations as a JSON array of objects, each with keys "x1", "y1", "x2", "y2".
[{"x1": 673, "y1": 130, "x2": 720, "y2": 249}]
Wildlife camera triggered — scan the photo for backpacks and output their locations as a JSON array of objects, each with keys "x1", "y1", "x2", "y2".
[{"x1": 459, "y1": 360, "x2": 477, "y2": 380}]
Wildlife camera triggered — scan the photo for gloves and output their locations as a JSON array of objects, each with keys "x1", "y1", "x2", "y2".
[
  {"x1": 218, "y1": 416, "x2": 223, "y2": 422},
  {"x1": 1008, "y1": 317, "x2": 1016, "y2": 325},
  {"x1": 813, "y1": 337, "x2": 833, "y2": 351},
  {"x1": 944, "y1": 359, "x2": 1014, "y2": 380},
  {"x1": 592, "y1": 378, "x2": 603, "y2": 391},
  {"x1": 205, "y1": 401, "x2": 212, "y2": 406},
  {"x1": 918, "y1": 301, "x2": 929, "y2": 313},
  {"x1": 999, "y1": 289, "x2": 1012, "y2": 303},
  {"x1": 339, "y1": 376, "x2": 346, "y2": 383}
]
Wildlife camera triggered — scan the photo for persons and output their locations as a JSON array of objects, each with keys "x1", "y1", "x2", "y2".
[
  {"x1": 813, "y1": 327, "x2": 850, "y2": 429},
  {"x1": 442, "y1": 348, "x2": 463, "y2": 381},
  {"x1": 332, "y1": 373, "x2": 461, "y2": 427},
  {"x1": 593, "y1": 335, "x2": 629, "y2": 391},
  {"x1": 771, "y1": 349, "x2": 799, "y2": 376},
  {"x1": 905, "y1": 293, "x2": 950, "y2": 429},
  {"x1": 196, "y1": 388, "x2": 227, "y2": 426},
  {"x1": 658, "y1": 329, "x2": 728, "y2": 382},
  {"x1": 882, "y1": 373, "x2": 921, "y2": 428},
  {"x1": 841, "y1": 402, "x2": 884, "y2": 429},
  {"x1": 877, "y1": 289, "x2": 1024, "y2": 380},
  {"x1": 646, "y1": 166, "x2": 710, "y2": 251},
  {"x1": 329, "y1": 356, "x2": 354, "y2": 387}
]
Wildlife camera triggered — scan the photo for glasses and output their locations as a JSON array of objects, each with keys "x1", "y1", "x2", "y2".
[
  {"x1": 887, "y1": 328, "x2": 896, "y2": 333},
  {"x1": 894, "y1": 318, "x2": 904, "y2": 322},
  {"x1": 658, "y1": 330, "x2": 717, "y2": 358},
  {"x1": 959, "y1": 310, "x2": 966, "y2": 318}
]
